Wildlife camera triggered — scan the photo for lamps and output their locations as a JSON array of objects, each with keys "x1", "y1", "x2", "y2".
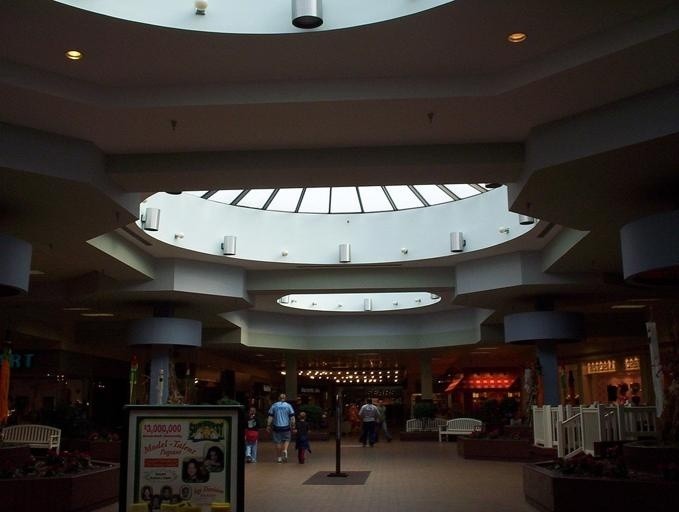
[
  {"x1": 364, "y1": 298, "x2": 371, "y2": 310},
  {"x1": 141, "y1": 207, "x2": 160, "y2": 230},
  {"x1": 450, "y1": 232, "x2": 466, "y2": 252},
  {"x1": 195, "y1": 1, "x2": 207, "y2": 16},
  {"x1": 340, "y1": 244, "x2": 350, "y2": 261},
  {"x1": 519, "y1": 214, "x2": 535, "y2": 225},
  {"x1": 292, "y1": 1, "x2": 322, "y2": 29},
  {"x1": 221, "y1": 235, "x2": 236, "y2": 255},
  {"x1": 281, "y1": 296, "x2": 289, "y2": 303},
  {"x1": 430, "y1": 292, "x2": 440, "y2": 299}
]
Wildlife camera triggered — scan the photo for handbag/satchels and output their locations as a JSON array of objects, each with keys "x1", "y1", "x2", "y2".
[{"x1": 245, "y1": 430, "x2": 259, "y2": 444}]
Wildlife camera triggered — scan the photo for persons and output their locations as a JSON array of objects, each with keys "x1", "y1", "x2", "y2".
[
  {"x1": 616, "y1": 387, "x2": 640, "y2": 403},
  {"x1": 266, "y1": 393, "x2": 296, "y2": 462},
  {"x1": 358, "y1": 398, "x2": 393, "y2": 448},
  {"x1": 246, "y1": 407, "x2": 260, "y2": 463},
  {"x1": 290, "y1": 412, "x2": 311, "y2": 464}
]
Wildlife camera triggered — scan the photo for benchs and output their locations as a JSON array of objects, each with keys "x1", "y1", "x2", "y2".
[
  {"x1": 438, "y1": 418, "x2": 483, "y2": 442},
  {"x1": 406, "y1": 417, "x2": 447, "y2": 433},
  {"x1": 1, "y1": 424, "x2": 61, "y2": 453}
]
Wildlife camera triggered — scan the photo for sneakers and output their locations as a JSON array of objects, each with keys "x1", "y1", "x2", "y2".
[
  {"x1": 247, "y1": 456, "x2": 256, "y2": 463},
  {"x1": 277, "y1": 450, "x2": 288, "y2": 462}
]
